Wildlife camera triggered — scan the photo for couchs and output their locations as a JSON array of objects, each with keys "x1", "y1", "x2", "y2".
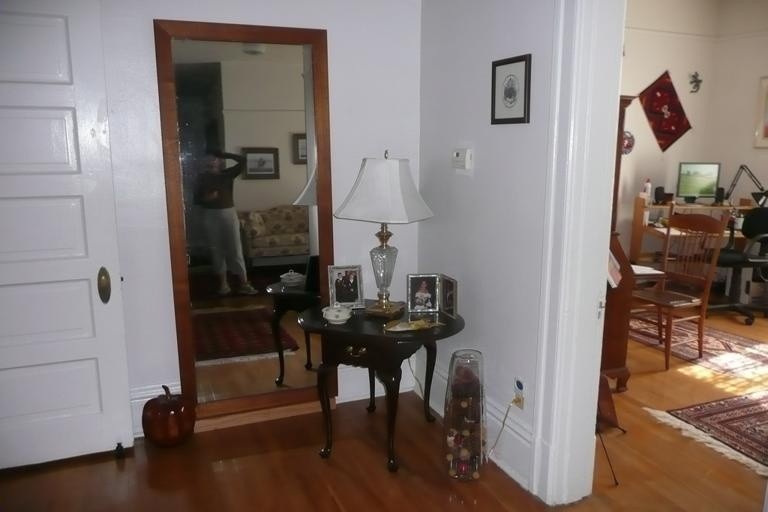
[{"x1": 237, "y1": 204, "x2": 308, "y2": 271}]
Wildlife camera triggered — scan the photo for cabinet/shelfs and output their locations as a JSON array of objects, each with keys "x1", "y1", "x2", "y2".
[
  {"x1": 629, "y1": 197, "x2": 758, "y2": 305},
  {"x1": 600, "y1": 94, "x2": 638, "y2": 392}
]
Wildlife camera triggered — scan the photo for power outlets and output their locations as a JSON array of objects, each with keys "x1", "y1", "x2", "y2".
[
  {"x1": 513, "y1": 393, "x2": 525, "y2": 409},
  {"x1": 450, "y1": 147, "x2": 470, "y2": 171}
]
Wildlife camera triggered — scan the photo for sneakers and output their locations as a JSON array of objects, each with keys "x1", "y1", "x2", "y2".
[
  {"x1": 218, "y1": 281, "x2": 232, "y2": 296},
  {"x1": 239, "y1": 281, "x2": 260, "y2": 295}
]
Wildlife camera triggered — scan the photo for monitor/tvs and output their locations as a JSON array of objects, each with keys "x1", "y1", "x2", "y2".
[{"x1": 676, "y1": 161, "x2": 721, "y2": 207}]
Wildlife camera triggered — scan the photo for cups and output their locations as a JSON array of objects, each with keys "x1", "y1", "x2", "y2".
[
  {"x1": 322, "y1": 302, "x2": 351, "y2": 325},
  {"x1": 281, "y1": 270, "x2": 303, "y2": 286}
]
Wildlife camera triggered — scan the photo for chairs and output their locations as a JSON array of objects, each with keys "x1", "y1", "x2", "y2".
[
  {"x1": 695, "y1": 206, "x2": 768, "y2": 325},
  {"x1": 630, "y1": 201, "x2": 728, "y2": 369}
]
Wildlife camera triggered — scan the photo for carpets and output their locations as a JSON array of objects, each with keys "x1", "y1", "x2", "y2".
[
  {"x1": 641, "y1": 386, "x2": 768, "y2": 479},
  {"x1": 629, "y1": 306, "x2": 768, "y2": 384}
]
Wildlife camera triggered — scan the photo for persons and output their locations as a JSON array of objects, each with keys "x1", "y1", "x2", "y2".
[
  {"x1": 415, "y1": 281, "x2": 432, "y2": 310},
  {"x1": 351, "y1": 272, "x2": 357, "y2": 291},
  {"x1": 345, "y1": 288, "x2": 355, "y2": 302},
  {"x1": 187, "y1": 150, "x2": 258, "y2": 295},
  {"x1": 335, "y1": 273, "x2": 344, "y2": 301},
  {"x1": 347, "y1": 274, "x2": 356, "y2": 291},
  {"x1": 342, "y1": 271, "x2": 349, "y2": 282}
]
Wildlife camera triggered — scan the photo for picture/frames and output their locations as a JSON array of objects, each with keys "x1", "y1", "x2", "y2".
[
  {"x1": 490, "y1": 54, "x2": 531, "y2": 125},
  {"x1": 753, "y1": 74, "x2": 768, "y2": 148},
  {"x1": 407, "y1": 274, "x2": 438, "y2": 313},
  {"x1": 241, "y1": 147, "x2": 279, "y2": 180},
  {"x1": 292, "y1": 132, "x2": 308, "y2": 163},
  {"x1": 439, "y1": 274, "x2": 457, "y2": 318},
  {"x1": 327, "y1": 264, "x2": 364, "y2": 309}
]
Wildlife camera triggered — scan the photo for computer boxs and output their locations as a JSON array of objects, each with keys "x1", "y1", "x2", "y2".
[{"x1": 731, "y1": 262, "x2": 753, "y2": 304}]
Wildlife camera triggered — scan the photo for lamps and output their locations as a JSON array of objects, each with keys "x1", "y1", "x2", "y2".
[
  {"x1": 240, "y1": 42, "x2": 266, "y2": 54},
  {"x1": 331, "y1": 147, "x2": 436, "y2": 320},
  {"x1": 725, "y1": 164, "x2": 768, "y2": 208}
]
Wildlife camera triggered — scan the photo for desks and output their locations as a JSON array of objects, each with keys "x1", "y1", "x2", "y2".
[{"x1": 297, "y1": 299, "x2": 465, "y2": 474}]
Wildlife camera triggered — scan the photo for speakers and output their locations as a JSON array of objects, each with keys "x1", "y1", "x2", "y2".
[
  {"x1": 715, "y1": 188, "x2": 724, "y2": 203},
  {"x1": 655, "y1": 187, "x2": 664, "y2": 203}
]
